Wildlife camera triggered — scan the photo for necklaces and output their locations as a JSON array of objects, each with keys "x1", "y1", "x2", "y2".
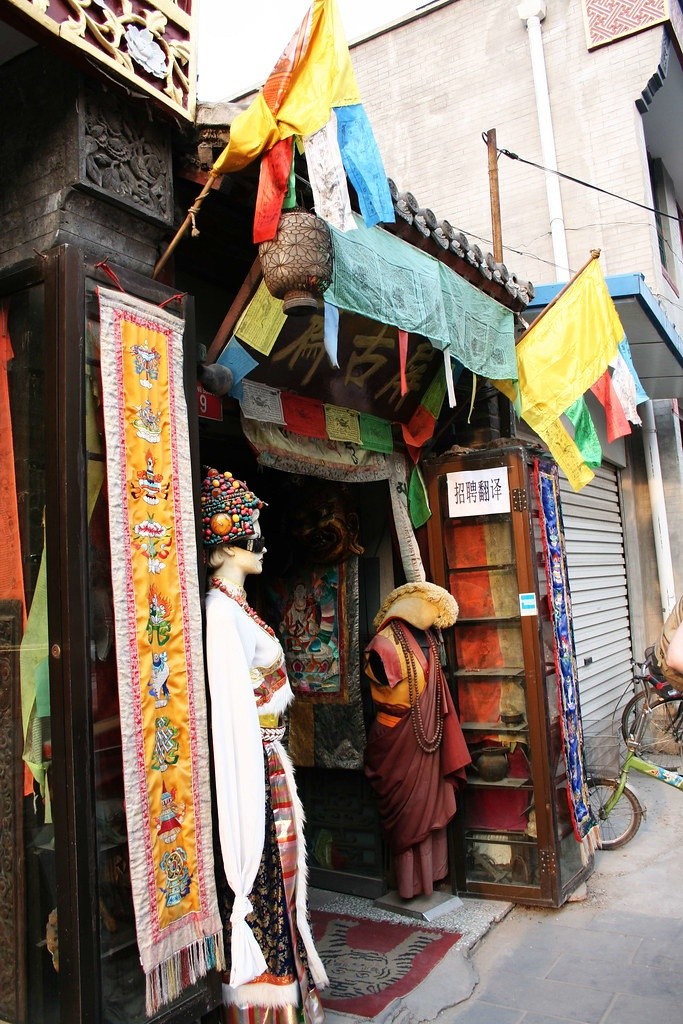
[{"x1": 388, "y1": 621, "x2": 445, "y2": 754}]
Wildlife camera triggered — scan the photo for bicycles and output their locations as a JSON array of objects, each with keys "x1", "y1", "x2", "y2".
[
  {"x1": 582, "y1": 658, "x2": 683, "y2": 852},
  {"x1": 620, "y1": 643, "x2": 683, "y2": 774}
]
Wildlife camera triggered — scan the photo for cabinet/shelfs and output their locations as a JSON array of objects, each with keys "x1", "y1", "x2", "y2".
[
  {"x1": 0, "y1": 244, "x2": 227, "y2": 1024},
  {"x1": 429, "y1": 448, "x2": 596, "y2": 910}
]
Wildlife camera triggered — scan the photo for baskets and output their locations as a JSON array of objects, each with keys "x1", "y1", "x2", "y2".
[{"x1": 582, "y1": 720, "x2": 622, "y2": 778}]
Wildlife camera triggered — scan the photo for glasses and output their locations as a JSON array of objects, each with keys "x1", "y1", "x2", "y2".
[{"x1": 226, "y1": 536, "x2": 264, "y2": 552}]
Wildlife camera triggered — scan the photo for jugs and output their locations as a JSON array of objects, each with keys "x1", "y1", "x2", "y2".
[{"x1": 470, "y1": 747, "x2": 511, "y2": 782}]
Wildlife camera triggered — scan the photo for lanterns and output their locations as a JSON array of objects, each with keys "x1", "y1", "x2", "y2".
[{"x1": 258, "y1": 206, "x2": 334, "y2": 315}]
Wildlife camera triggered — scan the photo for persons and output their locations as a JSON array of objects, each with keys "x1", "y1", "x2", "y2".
[
  {"x1": 200, "y1": 464, "x2": 328, "y2": 1024},
  {"x1": 364, "y1": 583, "x2": 471, "y2": 899}
]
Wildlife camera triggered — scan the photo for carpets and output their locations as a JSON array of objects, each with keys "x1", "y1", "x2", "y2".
[{"x1": 308, "y1": 909, "x2": 462, "y2": 1018}]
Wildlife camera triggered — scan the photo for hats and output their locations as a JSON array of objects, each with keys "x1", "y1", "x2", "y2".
[
  {"x1": 373, "y1": 582, "x2": 459, "y2": 632},
  {"x1": 201, "y1": 463, "x2": 263, "y2": 545}
]
